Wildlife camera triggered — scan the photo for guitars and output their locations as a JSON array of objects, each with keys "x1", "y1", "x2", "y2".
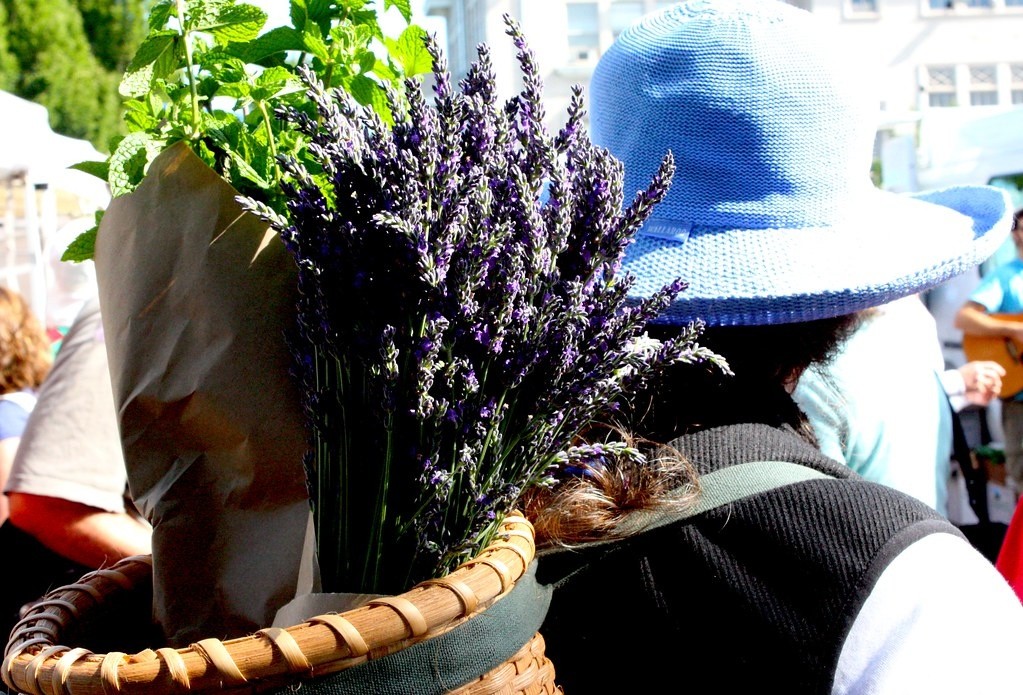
[{"x1": 962, "y1": 312, "x2": 1023, "y2": 400}]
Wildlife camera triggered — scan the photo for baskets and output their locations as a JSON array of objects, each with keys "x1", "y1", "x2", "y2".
[{"x1": 0, "y1": 504, "x2": 569, "y2": 695}]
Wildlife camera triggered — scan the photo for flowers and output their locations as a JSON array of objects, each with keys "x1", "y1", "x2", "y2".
[{"x1": 235, "y1": 13, "x2": 739, "y2": 591}]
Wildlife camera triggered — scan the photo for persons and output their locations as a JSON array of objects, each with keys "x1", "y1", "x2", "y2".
[
  {"x1": 0, "y1": 285, "x2": 151, "y2": 652},
  {"x1": 528, "y1": 0, "x2": 1023, "y2": 695}
]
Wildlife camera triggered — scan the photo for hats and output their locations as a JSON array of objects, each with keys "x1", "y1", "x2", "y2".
[{"x1": 536, "y1": 0, "x2": 1014, "y2": 327}]
